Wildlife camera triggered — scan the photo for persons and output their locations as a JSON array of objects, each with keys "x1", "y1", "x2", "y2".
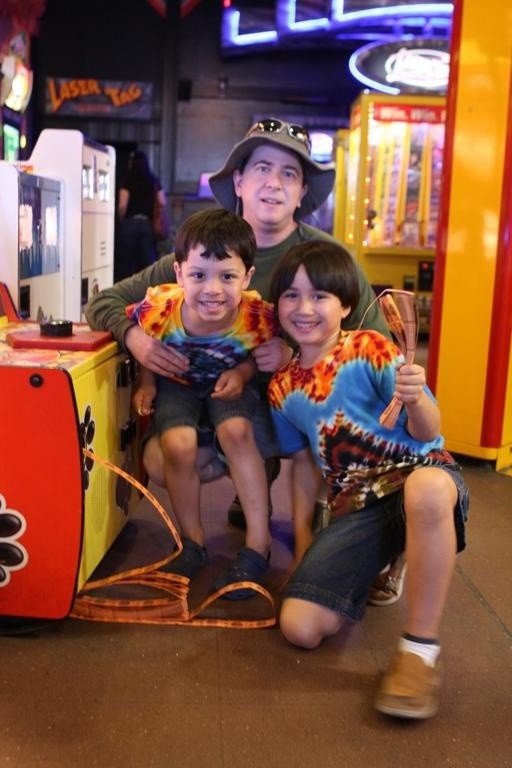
[
  {"x1": 265, "y1": 236, "x2": 472, "y2": 719},
  {"x1": 116, "y1": 150, "x2": 168, "y2": 284},
  {"x1": 121, "y1": 209, "x2": 285, "y2": 602},
  {"x1": 82, "y1": 114, "x2": 396, "y2": 535}
]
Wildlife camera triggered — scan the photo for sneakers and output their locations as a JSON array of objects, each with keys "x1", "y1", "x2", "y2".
[
  {"x1": 226, "y1": 457, "x2": 282, "y2": 528},
  {"x1": 366, "y1": 554, "x2": 408, "y2": 606},
  {"x1": 371, "y1": 651, "x2": 442, "y2": 719}
]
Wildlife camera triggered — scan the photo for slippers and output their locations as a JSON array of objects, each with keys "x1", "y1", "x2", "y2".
[
  {"x1": 162, "y1": 539, "x2": 209, "y2": 581},
  {"x1": 222, "y1": 547, "x2": 273, "y2": 590}
]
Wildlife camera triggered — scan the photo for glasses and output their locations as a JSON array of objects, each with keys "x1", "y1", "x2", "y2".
[{"x1": 248, "y1": 117, "x2": 311, "y2": 146}]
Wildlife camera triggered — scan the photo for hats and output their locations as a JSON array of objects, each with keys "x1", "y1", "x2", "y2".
[{"x1": 207, "y1": 118, "x2": 336, "y2": 221}]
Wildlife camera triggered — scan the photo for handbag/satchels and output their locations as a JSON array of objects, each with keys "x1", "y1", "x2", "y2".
[{"x1": 151, "y1": 174, "x2": 170, "y2": 242}]
